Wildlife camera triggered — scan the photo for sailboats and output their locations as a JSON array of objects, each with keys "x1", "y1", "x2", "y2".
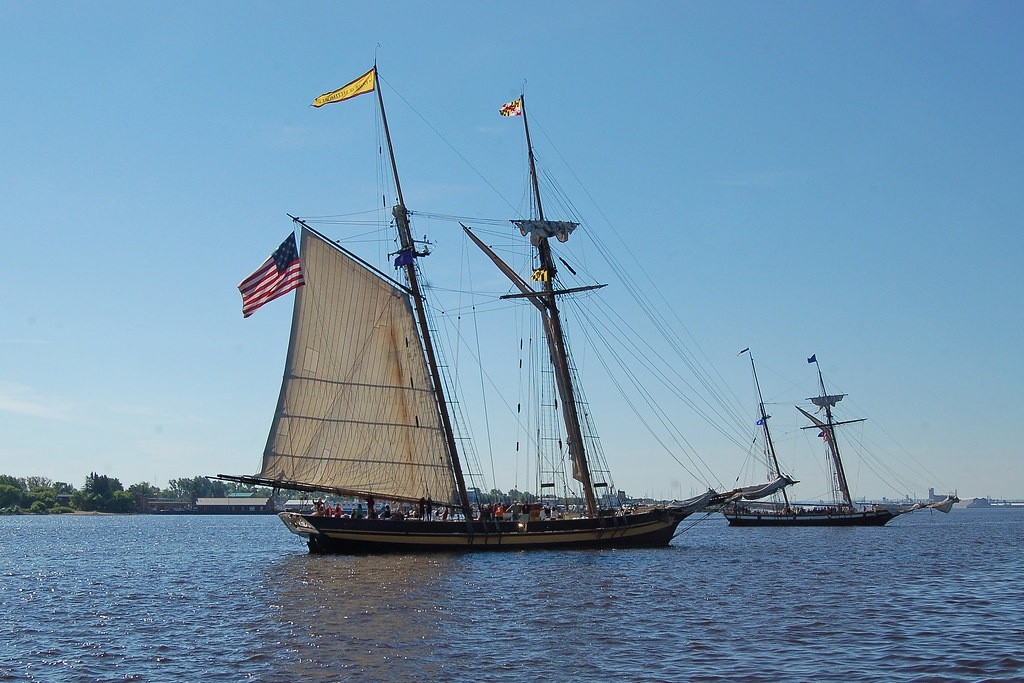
[
  {"x1": 721, "y1": 346, "x2": 964, "y2": 527},
  {"x1": 207, "y1": 38, "x2": 800, "y2": 552}
]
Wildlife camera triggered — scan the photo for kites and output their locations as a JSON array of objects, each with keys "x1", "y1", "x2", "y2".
[{"x1": 818, "y1": 429, "x2": 828, "y2": 442}]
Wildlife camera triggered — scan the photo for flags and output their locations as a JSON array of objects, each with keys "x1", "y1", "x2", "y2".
[
  {"x1": 757, "y1": 418, "x2": 764, "y2": 425},
  {"x1": 311, "y1": 65, "x2": 376, "y2": 108},
  {"x1": 806, "y1": 354, "x2": 816, "y2": 363},
  {"x1": 236, "y1": 232, "x2": 306, "y2": 318},
  {"x1": 499, "y1": 98, "x2": 524, "y2": 117},
  {"x1": 531, "y1": 270, "x2": 548, "y2": 281},
  {"x1": 393, "y1": 250, "x2": 413, "y2": 269}
]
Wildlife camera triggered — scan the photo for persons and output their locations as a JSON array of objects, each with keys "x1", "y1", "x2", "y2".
[
  {"x1": 722, "y1": 503, "x2": 877, "y2": 517},
  {"x1": 308, "y1": 495, "x2": 638, "y2": 523}
]
